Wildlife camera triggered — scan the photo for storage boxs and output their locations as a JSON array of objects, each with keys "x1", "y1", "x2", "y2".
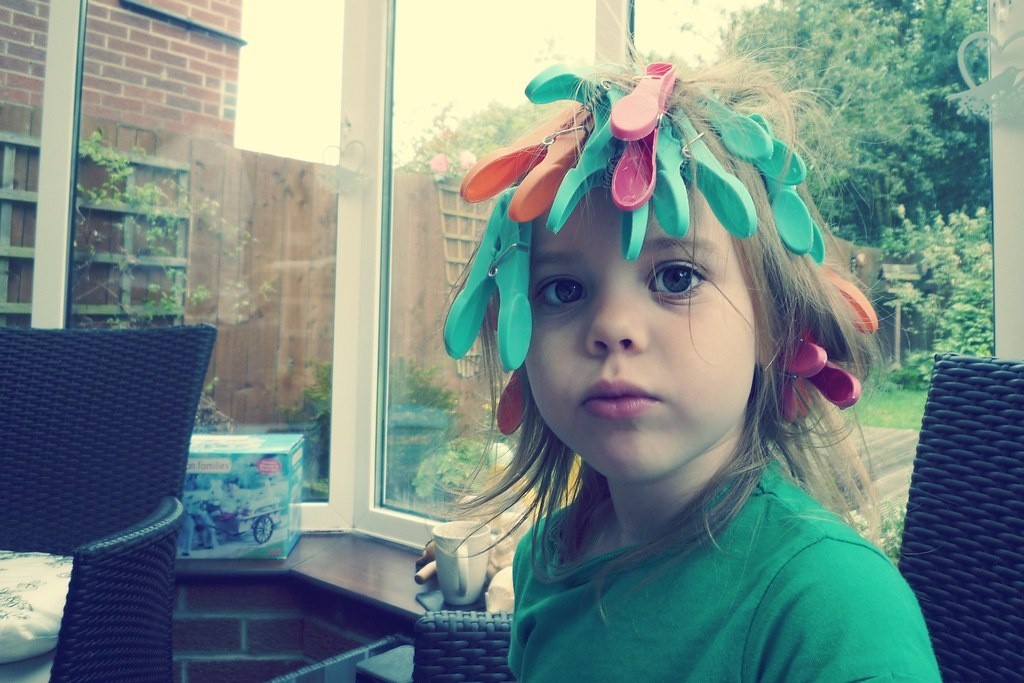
[{"x1": 175, "y1": 434, "x2": 306, "y2": 560}]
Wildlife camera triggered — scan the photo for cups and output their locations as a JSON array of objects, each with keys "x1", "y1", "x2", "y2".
[{"x1": 431, "y1": 520, "x2": 492, "y2": 606}]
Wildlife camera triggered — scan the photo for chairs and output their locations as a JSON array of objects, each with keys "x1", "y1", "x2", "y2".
[
  {"x1": 412, "y1": 352, "x2": 1024, "y2": 683},
  {"x1": 0, "y1": 323, "x2": 216, "y2": 683}
]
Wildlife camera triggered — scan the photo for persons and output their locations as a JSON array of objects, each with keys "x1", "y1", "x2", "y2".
[{"x1": 444, "y1": 64, "x2": 943, "y2": 683}]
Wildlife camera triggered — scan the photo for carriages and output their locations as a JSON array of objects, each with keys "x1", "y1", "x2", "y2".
[{"x1": 180, "y1": 487, "x2": 284, "y2": 557}]
